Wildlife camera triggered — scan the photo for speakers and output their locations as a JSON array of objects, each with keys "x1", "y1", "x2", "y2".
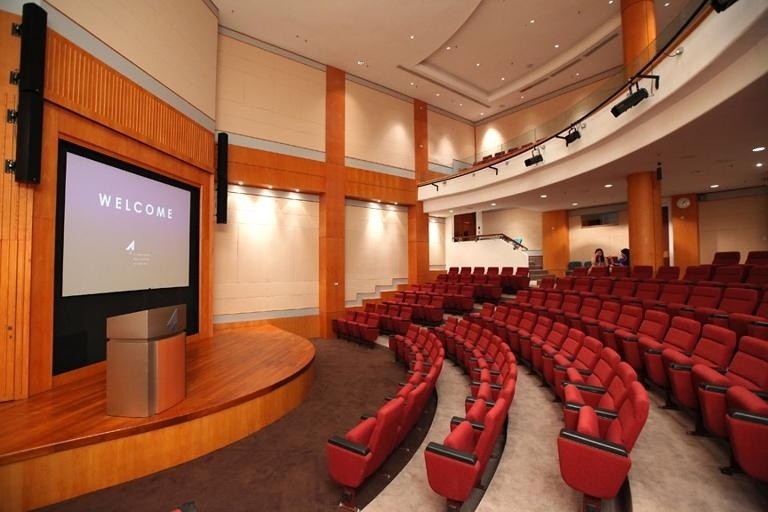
[
  {"x1": 524, "y1": 154, "x2": 543, "y2": 167},
  {"x1": 13, "y1": 3, "x2": 50, "y2": 184},
  {"x1": 216, "y1": 132, "x2": 230, "y2": 226},
  {"x1": 610, "y1": 87, "x2": 648, "y2": 119},
  {"x1": 565, "y1": 131, "x2": 580, "y2": 143}
]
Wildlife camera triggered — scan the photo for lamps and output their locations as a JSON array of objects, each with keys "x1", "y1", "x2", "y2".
[
  {"x1": 610, "y1": 74, "x2": 659, "y2": 118},
  {"x1": 555, "y1": 127, "x2": 580, "y2": 146},
  {"x1": 523, "y1": 147, "x2": 543, "y2": 166}
]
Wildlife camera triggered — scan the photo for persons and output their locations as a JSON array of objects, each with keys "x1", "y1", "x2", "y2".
[
  {"x1": 588, "y1": 248, "x2": 608, "y2": 275},
  {"x1": 609, "y1": 248, "x2": 629, "y2": 274}
]
